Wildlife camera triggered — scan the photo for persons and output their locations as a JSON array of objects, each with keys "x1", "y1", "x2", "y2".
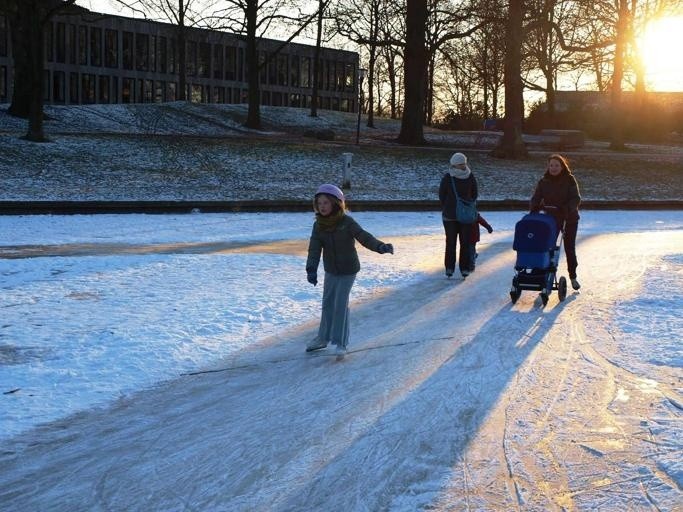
[
  {"x1": 305, "y1": 184, "x2": 394, "y2": 359},
  {"x1": 529, "y1": 154, "x2": 582, "y2": 290},
  {"x1": 471, "y1": 214, "x2": 493, "y2": 259},
  {"x1": 438, "y1": 152, "x2": 478, "y2": 279}
]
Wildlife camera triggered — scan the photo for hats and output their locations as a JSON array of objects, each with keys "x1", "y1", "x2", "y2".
[{"x1": 450, "y1": 152, "x2": 467, "y2": 167}]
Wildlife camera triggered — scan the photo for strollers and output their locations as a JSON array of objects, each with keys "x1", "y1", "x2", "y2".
[{"x1": 507, "y1": 205, "x2": 569, "y2": 311}]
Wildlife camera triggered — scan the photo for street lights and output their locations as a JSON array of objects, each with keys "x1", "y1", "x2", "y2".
[{"x1": 353, "y1": 67, "x2": 368, "y2": 144}]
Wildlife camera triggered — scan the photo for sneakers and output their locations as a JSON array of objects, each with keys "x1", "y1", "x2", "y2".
[
  {"x1": 571, "y1": 277, "x2": 580, "y2": 290},
  {"x1": 307, "y1": 336, "x2": 327, "y2": 349},
  {"x1": 445, "y1": 268, "x2": 453, "y2": 276},
  {"x1": 335, "y1": 344, "x2": 346, "y2": 356},
  {"x1": 460, "y1": 268, "x2": 469, "y2": 276}
]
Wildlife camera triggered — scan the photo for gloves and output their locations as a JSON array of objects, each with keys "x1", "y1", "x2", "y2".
[
  {"x1": 379, "y1": 242, "x2": 394, "y2": 255},
  {"x1": 306, "y1": 271, "x2": 318, "y2": 286},
  {"x1": 553, "y1": 204, "x2": 568, "y2": 218},
  {"x1": 487, "y1": 226, "x2": 493, "y2": 233},
  {"x1": 530, "y1": 200, "x2": 544, "y2": 212}
]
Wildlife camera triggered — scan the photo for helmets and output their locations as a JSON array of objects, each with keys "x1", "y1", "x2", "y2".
[{"x1": 314, "y1": 183, "x2": 345, "y2": 205}]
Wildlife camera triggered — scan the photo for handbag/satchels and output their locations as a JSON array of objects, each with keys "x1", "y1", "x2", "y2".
[{"x1": 455, "y1": 199, "x2": 479, "y2": 224}]
